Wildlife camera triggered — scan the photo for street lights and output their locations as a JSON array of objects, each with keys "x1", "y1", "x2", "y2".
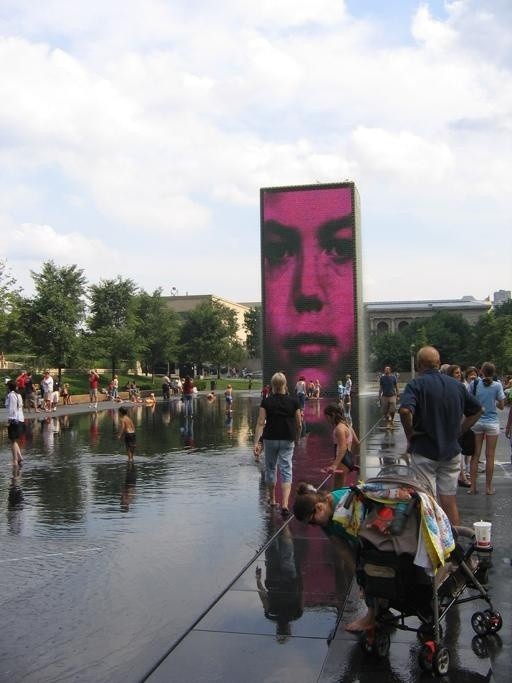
[{"x1": 408, "y1": 342, "x2": 416, "y2": 379}]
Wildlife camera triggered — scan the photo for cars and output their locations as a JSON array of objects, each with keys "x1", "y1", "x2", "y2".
[{"x1": 243, "y1": 370, "x2": 264, "y2": 378}]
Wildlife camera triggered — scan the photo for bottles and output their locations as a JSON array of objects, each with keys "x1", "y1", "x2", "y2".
[{"x1": 371, "y1": 502, "x2": 407, "y2": 534}]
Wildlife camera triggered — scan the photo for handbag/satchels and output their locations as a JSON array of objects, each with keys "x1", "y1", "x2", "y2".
[{"x1": 457, "y1": 427, "x2": 477, "y2": 456}]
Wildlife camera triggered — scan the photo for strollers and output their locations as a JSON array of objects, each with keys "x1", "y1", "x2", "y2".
[{"x1": 354, "y1": 461, "x2": 505, "y2": 677}]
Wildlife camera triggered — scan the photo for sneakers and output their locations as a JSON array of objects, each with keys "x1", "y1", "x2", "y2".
[
  {"x1": 476, "y1": 465, "x2": 486, "y2": 473},
  {"x1": 44, "y1": 406, "x2": 56, "y2": 412},
  {"x1": 88, "y1": 403, "x2": 93, "y2": 407},
  {"x1": 34, "y1": 408, "x2": 40, "y2": 412},
  {"x1": 93, "y1": 402, "x2": 97, "y2": 408}
]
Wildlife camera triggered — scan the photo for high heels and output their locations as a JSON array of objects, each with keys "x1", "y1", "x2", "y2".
[{"x1": 458, "y1": 478, "x2": 472, "y2": 487}]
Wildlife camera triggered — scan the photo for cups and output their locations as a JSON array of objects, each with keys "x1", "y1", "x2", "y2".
[{"x1": 473, "y1": 520, "x2": 493, "y2": 550}]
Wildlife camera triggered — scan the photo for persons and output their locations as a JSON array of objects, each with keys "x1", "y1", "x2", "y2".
[{"x1": 262, "y1": 185, "x2": 356, "y2": 400}]
